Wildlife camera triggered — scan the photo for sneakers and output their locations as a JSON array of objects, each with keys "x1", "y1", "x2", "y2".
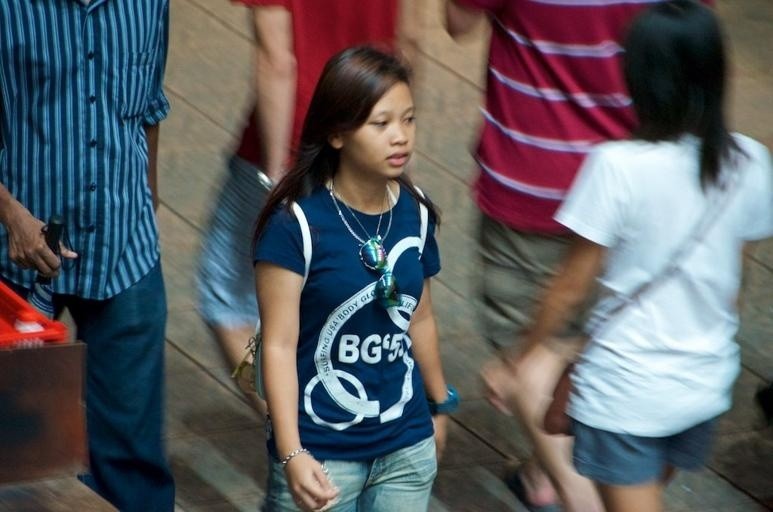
[{"x1": 509, "y1": 468, "x2": 565, "y2": 512}]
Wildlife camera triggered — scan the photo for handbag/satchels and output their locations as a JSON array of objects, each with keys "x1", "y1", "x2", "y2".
[
  {"x1": 543, "y1": 361, "x2": 576, "y2": 435},
  {"x1": 255, "y1": 319, "x2": 267, "y2": 398}
]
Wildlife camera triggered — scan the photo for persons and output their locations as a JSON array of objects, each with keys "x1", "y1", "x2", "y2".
[
  {"x1": 477, "y1": 1, "x2": 772, "y2": 510},
  {"x1": 249, "y1": 40, "x2": 462, "y2": 511},
  {"x1": 195, "y1": 1, "x2": 425, "y2": 419},
  {"x1": 434, "y1": 2, "x2": 721, "y2": 512},
  {"x1": 0, "y1": 0, "x2": 175, "y2": 511}
]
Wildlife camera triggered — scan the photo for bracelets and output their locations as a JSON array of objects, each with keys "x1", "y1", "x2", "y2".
[
  {"x1": 279, "y1": 448, "x2": 312, "y2": 463},
  {"x1": 426, "y1": 383, "x2": 460, "y2": 416}
]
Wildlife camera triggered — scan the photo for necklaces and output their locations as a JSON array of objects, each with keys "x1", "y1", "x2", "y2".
[{"x1": 322, "y1": 177, "x2": 400, "y2": 246}]
